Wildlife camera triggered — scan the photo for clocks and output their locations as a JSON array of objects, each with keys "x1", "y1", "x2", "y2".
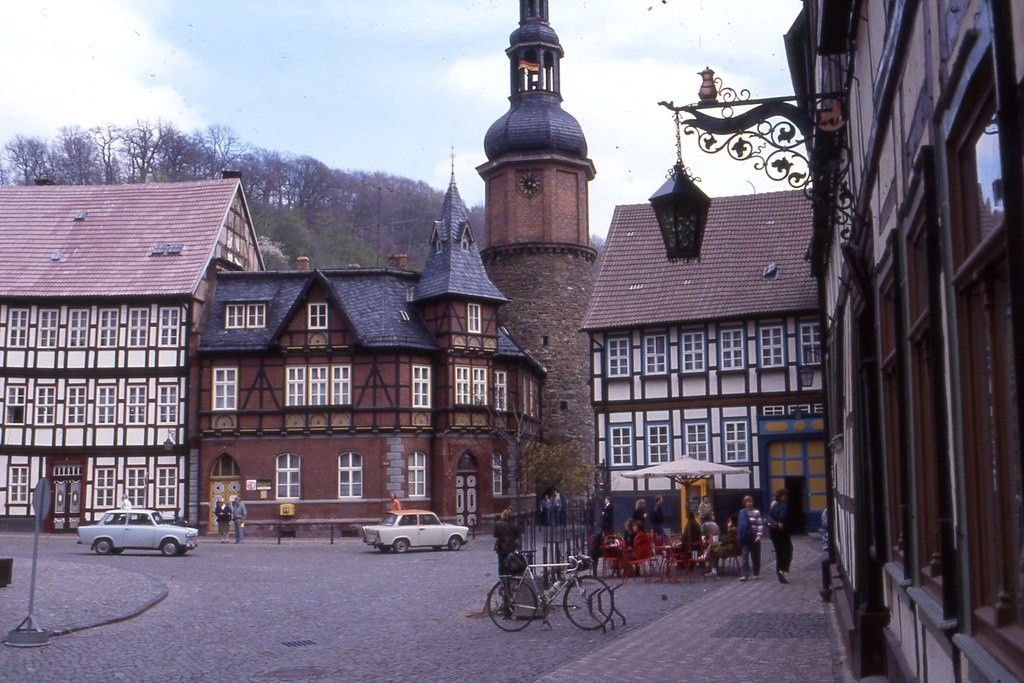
[{"x1": 518, "y1": 170, "x2": 542, "y2": 198}]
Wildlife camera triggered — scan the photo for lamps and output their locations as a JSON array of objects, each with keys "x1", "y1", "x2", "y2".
[
  {"x1": 798, "y1": 347, "x2": 823, "y2": 387},
  {"x1": 163, "y1": 429, "x2": 178, "y2": 451},
  {"x1": 649, "y1": 90, "x2": 850, "y2": 264}
]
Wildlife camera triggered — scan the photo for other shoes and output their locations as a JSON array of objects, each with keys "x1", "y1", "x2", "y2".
[
  {"x1": 740, "y1": 576, "x2": 747, "y2": 582},
  {"x1": 778, "y1": 571, "x2": 789, "y2": 584},
  {"x1": 705, "y1": 570, "x2": 718, "y2": 578},
  {"x1": 753, "y1": 576, "x2": 758, "y2": 580}
]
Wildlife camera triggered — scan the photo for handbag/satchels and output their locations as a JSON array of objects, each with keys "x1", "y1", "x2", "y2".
[{"x1": 765, "y1": 516, "x2": 778, "y2": 529}]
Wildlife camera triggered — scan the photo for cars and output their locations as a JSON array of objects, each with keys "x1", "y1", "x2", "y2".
[
  {"x1": 361, "y1": 509, "x2": 469, "y2": 555},
  {"x1": 77, "y1": 508, "x2": 199, "y2": 557}
]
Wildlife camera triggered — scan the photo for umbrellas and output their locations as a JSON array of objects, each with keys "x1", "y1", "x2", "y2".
[{"x1": 620, "y1": 455, "x2": 752, "y2": 543}]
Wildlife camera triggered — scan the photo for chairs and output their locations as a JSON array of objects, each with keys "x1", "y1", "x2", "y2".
[{"x1": 588, "y1": 529, "x2": 742, "y2": 585}]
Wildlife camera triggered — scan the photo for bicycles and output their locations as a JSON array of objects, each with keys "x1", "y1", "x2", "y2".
[{"x1": 486, "y1": 550, "x2": 615, "y2": 633}]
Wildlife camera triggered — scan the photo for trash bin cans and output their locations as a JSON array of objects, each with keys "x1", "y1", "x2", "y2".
[{"x1": 0, "y1": 557, "x2": 13, "y2": 588}]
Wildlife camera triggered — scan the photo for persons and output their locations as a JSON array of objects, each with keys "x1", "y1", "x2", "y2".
[
  {"x1": 540, "y1": 490, "x2": 562, "y2": 528},
  {"x1": 233, "y1": 497, "x2": 247, "y2": 544},
  {"x1": 387, "y1": 493, "x2": 401, "y2": 525},
  {"x1": 593, "y1": 495, "x2": 763, "y2": 580},
  {"x1": 768, "y1": 489, "x2": 793, "y2": 583},
  {"x1": 215, "y1": 497, "x2": 232, "y2": 542},
  {"x1": 494, "y1": 509, "x2": 521, "y2": 620}
]
